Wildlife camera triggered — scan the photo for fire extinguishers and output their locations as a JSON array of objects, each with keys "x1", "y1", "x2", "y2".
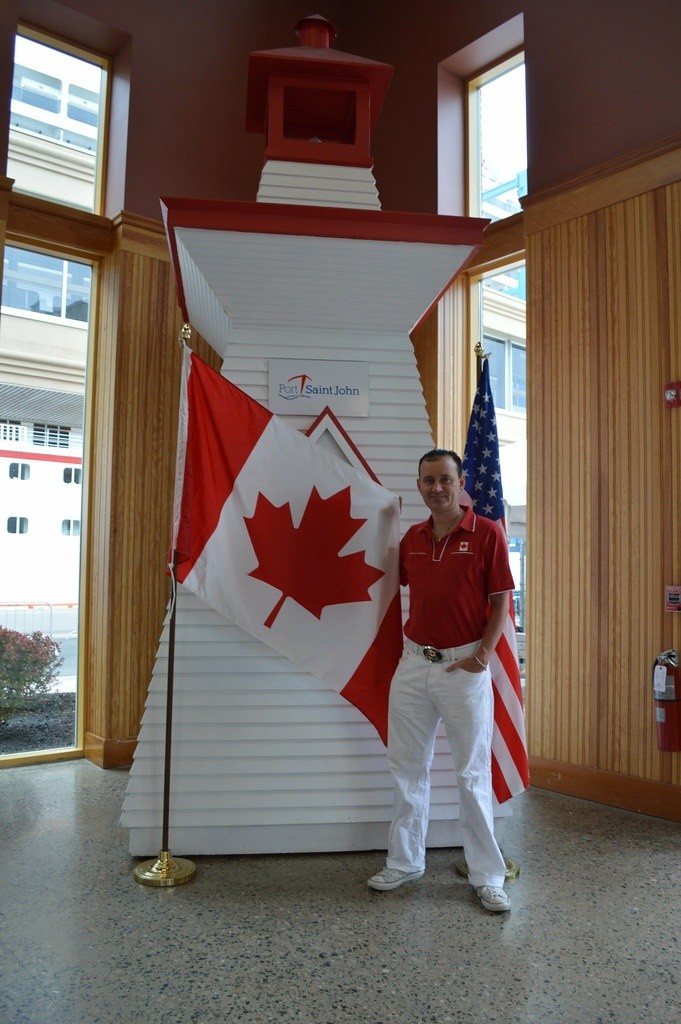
[{"x1": 650, "y1": 648, "x2": 681, "y2": 753}]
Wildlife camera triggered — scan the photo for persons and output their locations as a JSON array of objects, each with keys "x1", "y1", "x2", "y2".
[{"x1": 366, "y1": 449, "x2": 515, "y2": 913}]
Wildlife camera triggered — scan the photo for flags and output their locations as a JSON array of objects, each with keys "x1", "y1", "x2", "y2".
[
  {"x1": 461, "y1": 358, "x2": 531, "y2": 804},
  {"x1": 168, "y1": 344, "x2": 405, "y2": 748}
]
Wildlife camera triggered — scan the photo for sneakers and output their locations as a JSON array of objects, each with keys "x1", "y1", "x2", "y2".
[
  {"x1": 476, "y1": 883, "x2": 512, "y2": 912},
  {"x1": 366, "y1": 865, "x2": 426, "y2": 891}
]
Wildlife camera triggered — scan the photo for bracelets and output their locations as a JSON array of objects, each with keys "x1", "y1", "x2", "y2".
[{"x1": 473, "y1": 655, "x2": 488, "y2": 672}]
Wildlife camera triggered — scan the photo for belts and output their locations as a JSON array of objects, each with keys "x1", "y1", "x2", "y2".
[{"x1": 404, "y1": 638, "x2": 482, "y2": 664}]
